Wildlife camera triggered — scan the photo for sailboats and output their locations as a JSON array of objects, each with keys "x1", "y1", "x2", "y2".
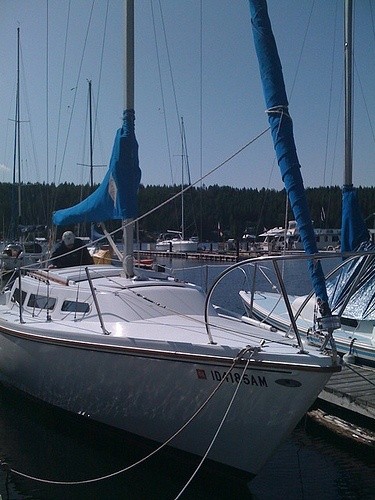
[
  {"x1": 0, "y1": 1, "x2": 346, "y2": 480},
  {"x1": 239, "y1": 0, "x2": 375, "y2": 455},
  {"x1": 156, "y1": 116, "x2": 349, "y2": 256},
  {"x1": 1, "y1": 27, "x2": 58, "y2": 271},
  {"x1": 65, "y1": 81, "x2": 176, "y2": 280}
]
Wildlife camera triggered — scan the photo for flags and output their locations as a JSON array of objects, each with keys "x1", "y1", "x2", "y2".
[{"x1": 133, "y1": 256, "x2": 154, "y2": 269}]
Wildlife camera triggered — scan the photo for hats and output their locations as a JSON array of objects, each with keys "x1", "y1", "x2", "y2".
[{"x1": 62, "y1": 231, "x2": 76, "y2": 245}]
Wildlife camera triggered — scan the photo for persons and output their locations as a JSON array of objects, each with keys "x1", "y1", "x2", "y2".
[{"x1": 48, "y1": 231, "x2": 94, "y2": 268}]
